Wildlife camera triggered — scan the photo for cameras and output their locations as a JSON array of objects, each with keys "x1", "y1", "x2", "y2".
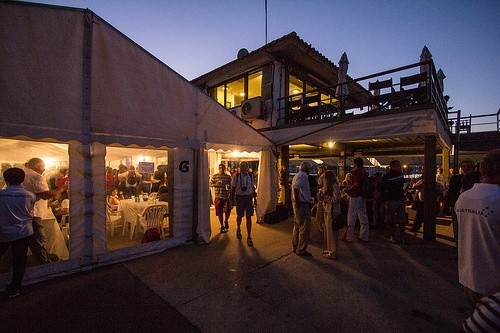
[{"x1": 242, "y1": 187, "x2": 247, "y2": 192}]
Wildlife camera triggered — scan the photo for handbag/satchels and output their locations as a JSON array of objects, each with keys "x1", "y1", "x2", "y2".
[
  {"x1": 333, "y1": 215, "x2": 346, "y2": 228},
  {"x1": 142, "y1": 227, "x2": 160, "y2": 243},
  {"x1": 265, "y1": 211, "x2": 279, "y2": 224}
]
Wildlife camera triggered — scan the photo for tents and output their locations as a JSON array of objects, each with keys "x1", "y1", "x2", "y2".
[
  {"x1": 260, "y1": 109, "x2": 453, "y2": 209},
  {"x1": 0, "y1": 0, "x2": 279, "y2": 290}
]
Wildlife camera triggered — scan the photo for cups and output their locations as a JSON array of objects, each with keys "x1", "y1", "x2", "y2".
[
  {"x1": 310, "y1": 197, "x2": 314, "y2": 204},
  {"x1": 131, "y1": 194, "x2": 143, "y2": 203},
  {"x1": 226, "y1": 184, "x2": 230, "y2": 190}
]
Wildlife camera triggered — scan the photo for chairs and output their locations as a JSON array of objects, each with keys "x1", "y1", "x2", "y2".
[
  {"x1": 106, "y1": 195, "x2": 126, "y2": 239},
  {"x1": 368, "y1": 70, "x2": 430, "y2": 112},
  {"x1": 129, "y1": 204, "x2": 168, "y2": 245},
  {"x1": 288, "y1": 91, "x2": 337, "y2": 124}
]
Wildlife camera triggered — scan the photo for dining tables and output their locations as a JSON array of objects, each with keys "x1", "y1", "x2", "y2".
[{"x1": 119, "y1": 198, "x2": 168, "y2": 240}]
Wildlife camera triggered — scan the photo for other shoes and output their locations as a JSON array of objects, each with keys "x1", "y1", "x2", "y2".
[
  {"x1": 221, "y1": 226, "x2": 224, "y2": 233},
  {"x1": 237, "y1": 230, "x2": 242, "y2": 238},
  {"x1": 224, "y1": 226, "x2": 229, "y2": 232},
  {"x1": 5, "y1": 284, "x2": 20, "y2": 298},
  {"x1": 401, "y1": 240, "x2": 406, "y2": 244},
  {"x1": 357, "y1": 238, "x2": 368, "y2": 243},
  {"x1": 299, "y1": 251, "x2": 312, "y2": 257},
  {"x1": 247, "y1": 238, "x2": 253, "y2": 247}
]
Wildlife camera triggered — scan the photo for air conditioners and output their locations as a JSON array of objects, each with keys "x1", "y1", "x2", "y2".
[{"x1": 238, "y1": 96, "x2": 266, "y2": 122}]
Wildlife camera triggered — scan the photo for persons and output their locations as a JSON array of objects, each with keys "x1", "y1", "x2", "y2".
[
  {"x1": 210, "y1": 163, "x2": 233, "y2": 233},
  {"x1": 287, "y1": 157, "x2": 446, "y2": 261},
  {"x1": 453, "y1": 150, "x2": 500, "y2": 319},
  {"x1": 46, "y1": 161, "x2": 172, "y2": 205},
  {"x1": 0, "y1": 167, "x2": 37, "y2": 298},
  {"x1": 461, "y1": 292, "x2": 500, "y2": 333},
  {"x1": 2, "y1": 158, "x2": 69, "y2": 268},
  {"x1": 229, "y1": 161, "x2": 257, "y2": 247},
  {"x1": 448, "y1": 158, "x2": 483, "y2": 248}
]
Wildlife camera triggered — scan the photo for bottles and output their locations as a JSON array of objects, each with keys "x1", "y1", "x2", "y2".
[{"x1": 143, "y1": 193, "x2": 148, "y2": 201}]
